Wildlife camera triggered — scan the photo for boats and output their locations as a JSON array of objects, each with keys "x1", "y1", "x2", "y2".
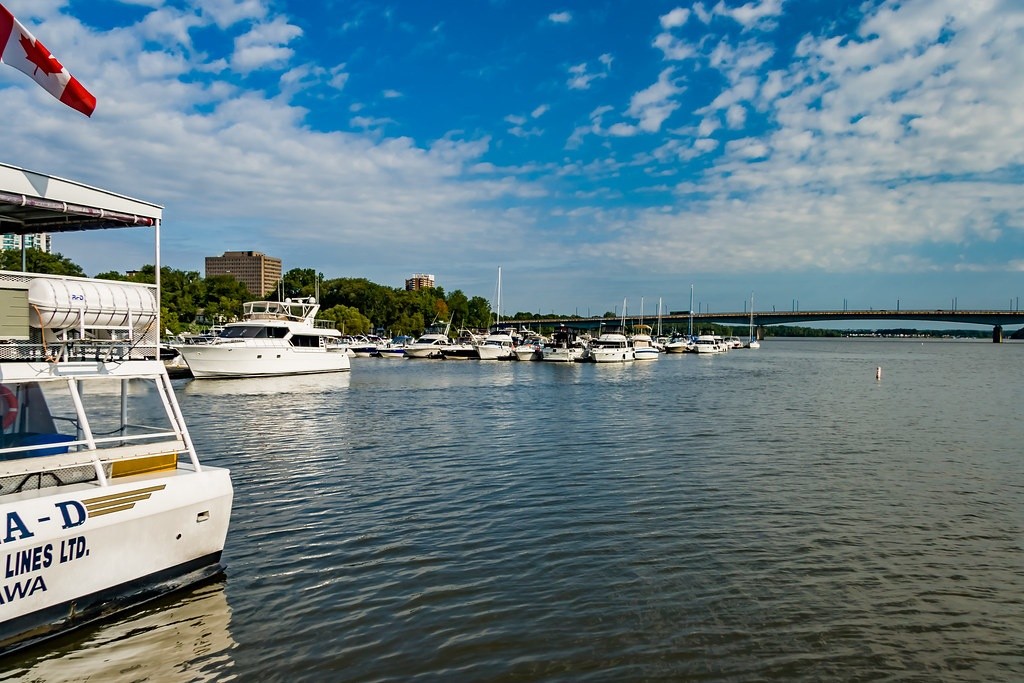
[
  {"x1": 326, "y1": 267, "x2": 743, "y2": 363},
  {"x1": 0, "y1": 160, "x2": 235, "y2": 644},
  {"x1": 162, "y1": 277, "x2": 351, "y2": 378}
]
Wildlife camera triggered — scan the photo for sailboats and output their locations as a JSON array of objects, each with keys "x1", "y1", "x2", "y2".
[{"x1": 746, "y1": 289, "x2": 761, "y2": 349}]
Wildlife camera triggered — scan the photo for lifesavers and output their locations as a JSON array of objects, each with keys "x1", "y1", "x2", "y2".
[{"x1": 0, "y1": 385, "x2": 19, "y2": 429}]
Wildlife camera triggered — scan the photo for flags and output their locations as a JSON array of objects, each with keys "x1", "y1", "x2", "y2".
[{"x1": 0, "y1": 3, "x2": 96, "y2": 117}]
